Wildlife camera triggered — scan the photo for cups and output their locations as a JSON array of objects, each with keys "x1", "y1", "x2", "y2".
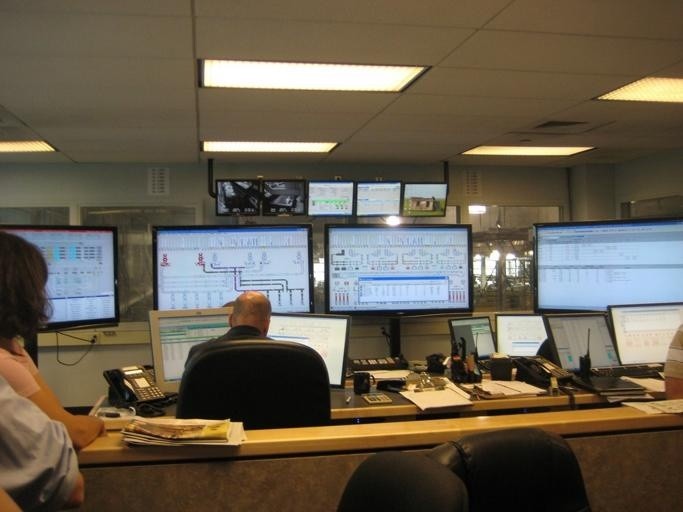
[
  {"x1": 425, "y1": 353, "x2": 446, "y2": 376},
  {"x1": 353, "y1": 371, "x2": 375, "y2": 395}
]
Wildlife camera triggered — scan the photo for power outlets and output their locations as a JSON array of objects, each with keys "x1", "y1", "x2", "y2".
[{"x1": 90, "y1": 332, "x2": 99, "y2": 346}]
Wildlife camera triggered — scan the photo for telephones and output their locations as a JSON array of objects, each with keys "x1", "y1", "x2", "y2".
[
  {"x1": 513, "y1": 355, "x2": 573, "y2": 386},
  {"x1": 103, "y1": 365, "x2": 165, "y2": 406}
]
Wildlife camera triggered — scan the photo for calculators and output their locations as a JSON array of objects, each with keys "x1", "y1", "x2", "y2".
[{"x1": 362, "y1": 393, "x2": 392, "y2": 404}]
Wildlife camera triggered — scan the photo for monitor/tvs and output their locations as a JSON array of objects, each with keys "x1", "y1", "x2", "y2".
[
  {"x1": 310, "y1": 180, "x2": 354, "y2": 218},
  {"x1": 215, "y1": 178, "x2": 260, "y2": 217},
  {"x1": 495, "y1": 312, "x2": 546, "y2": 357},
  {"x1": 150, "y1": 226, "x2": 315, "y2": 315},
  {"x1": 146, "y1": 306, "x2": 232, "y2": 394},
  {"x1": 324, "y1": 222, "x2": 475, "y2": 356},
  {"x1": 607, "y1": 302, "x2": 682, "y2": 365},
  {"x1": 541, "y1": 311, "x2": 622, "y2": 372},
  {"x1": 2, "y1": 223, "x2": 121, "y2": 330},
  {"x1": 356, "y1": 181, "x2": 402, "y2": 218},
  {"x1": 448, "y1": 316, "x2": 497, "y2": 360},
  {"x1": 266, "y1": 312, "x2": 352, "y2": 389},
  {"x1": 403, "y1": 181, "x2": 448, "y2": 219},
  {"x1": 262, "y1": 178, "x2": 307, "y2": 218},
  {"x1": 534, "y1": 218, "x2": 682, "y2": 312}
]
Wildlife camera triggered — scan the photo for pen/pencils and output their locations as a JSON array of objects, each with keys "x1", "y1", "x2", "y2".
[{"x1": 346, "y1": 396, "x2": 352, "y2": 402}]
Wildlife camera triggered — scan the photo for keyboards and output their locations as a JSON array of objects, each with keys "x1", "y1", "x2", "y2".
[{"x1": 593, "y1": 365, "x2": 657, "y2": 381}]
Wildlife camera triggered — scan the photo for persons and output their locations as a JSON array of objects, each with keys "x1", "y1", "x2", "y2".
[
  {"x1": 1, "y1": 231, "x2": 104, "y2": 450},
  {"x1": 664, "y1": 324, "x2": 683, "y2": 399},
  {"x1": 187, "y1": 290, "x2": 274, "y2": 364},
  {"x1": 0, "y1": 376, "x2": 84, "y2": 512}
]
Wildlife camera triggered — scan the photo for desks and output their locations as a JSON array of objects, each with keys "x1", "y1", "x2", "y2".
[{"x1": 88, "y1": 356, "x2": 665, "y2": 430}]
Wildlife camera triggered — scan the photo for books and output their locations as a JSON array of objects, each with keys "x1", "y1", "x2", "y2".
[
  {"x1": 571, "y1": 376, "x2": 646, "y2": 396},
  {"x1": 460, "y1": 380, "x2": 547, "y2": 399},
  {"x1": 359, "y1": 369, "x2": 412, "y2": 381}
]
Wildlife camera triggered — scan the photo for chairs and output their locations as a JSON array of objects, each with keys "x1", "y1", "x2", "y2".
[
  {"x1": 429, "y1": 430, "x2": 591, "y2": 511},
  {"x1": 336, "y1": 450, "x2": 469, "y2": 511}
]
